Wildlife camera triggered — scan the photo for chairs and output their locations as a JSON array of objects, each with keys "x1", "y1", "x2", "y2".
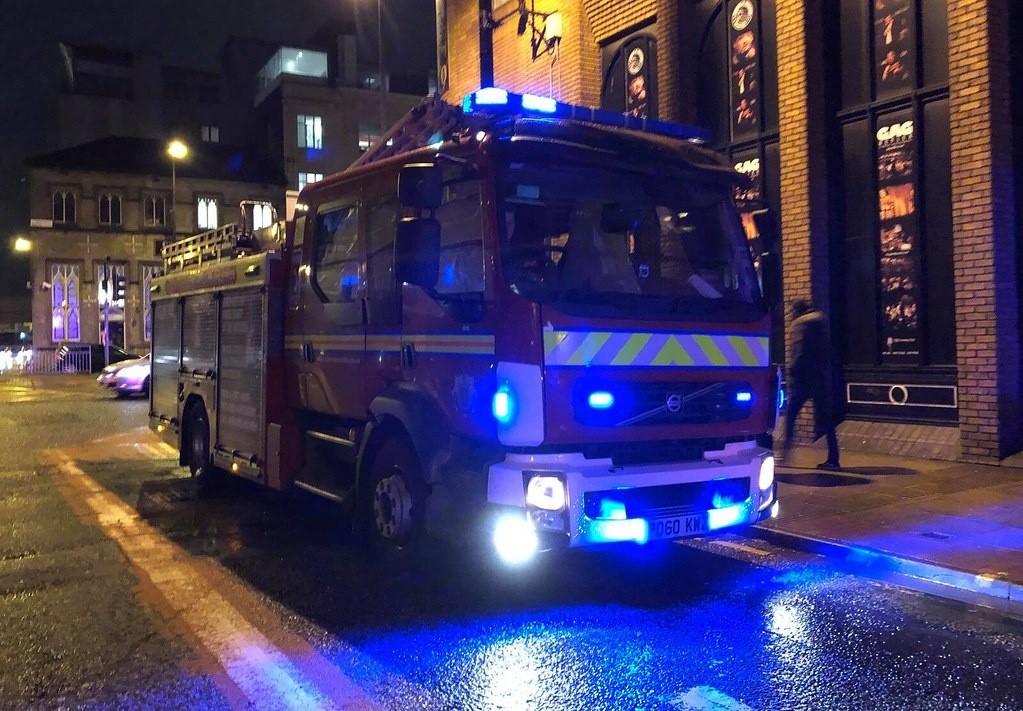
[{"x1": 561, "y1": 211, "x2": 643, "y2": 297}]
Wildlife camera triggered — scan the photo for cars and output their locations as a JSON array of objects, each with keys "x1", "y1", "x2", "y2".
[
  {"x1": 98, "y1": 353, "x2": 151, "y2": 400},
  {"x1": 53, "y1": 344, "x2": 142, "y2": 374}
]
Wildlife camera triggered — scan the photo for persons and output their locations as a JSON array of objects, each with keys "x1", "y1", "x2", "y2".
[{"x1": 778, "y1": 297, "x2": 847, "y2": 471}]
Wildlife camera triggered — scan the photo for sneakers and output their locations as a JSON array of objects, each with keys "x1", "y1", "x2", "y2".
[
  {"x1": 775, "y1": 457, "x2": 789, "y2": 467},
  {"x1": 816, "y1": 460, "x2": 841, "y2": 471}
]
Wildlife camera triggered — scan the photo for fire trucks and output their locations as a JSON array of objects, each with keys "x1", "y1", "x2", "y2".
[{"x1": 148, "y1": 88, "x2": 782, "y2": 565}]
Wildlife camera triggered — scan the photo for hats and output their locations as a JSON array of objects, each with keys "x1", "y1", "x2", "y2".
[{"x1": 792, "y1": 301, "x2": 813, "y2": 318}]
